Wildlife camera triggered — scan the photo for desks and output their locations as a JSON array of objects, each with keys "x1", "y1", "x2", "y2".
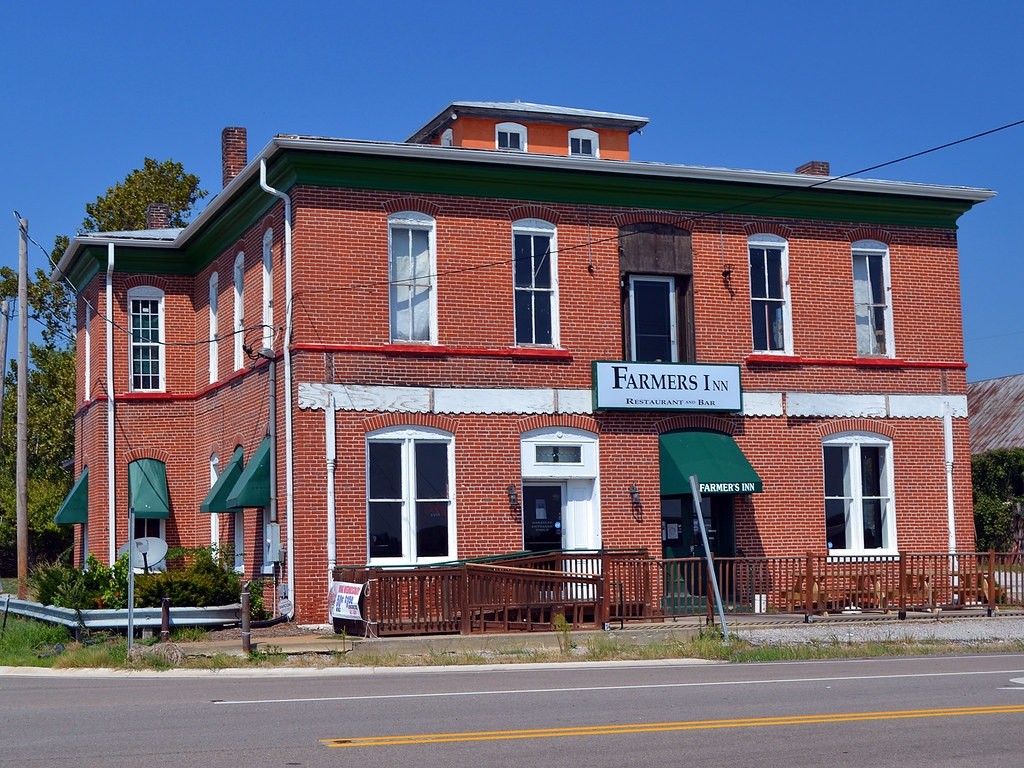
[
  {"x1": 780, "y1": 570, "x2": 892, "y2": 615},
  {"x1": 892, "y1": 567, "x2": 1002, "y2": 612}
]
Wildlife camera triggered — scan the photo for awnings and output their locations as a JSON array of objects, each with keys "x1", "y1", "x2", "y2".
[
  {"x1": 54, "y1": 466, "x2": 88, "y2": 524},
  {"x1": 659, "y1": 428, "x2": 762, "y2": 495},
  {"x1": 226, "y1": 434, "x2": 271, "y2": 508},
  {"x1": 199, "y1": 447, "x2": 243, "y2": 513},
  {"x1": 128, "y1": 458, "x2": 170, "y2": 519}
]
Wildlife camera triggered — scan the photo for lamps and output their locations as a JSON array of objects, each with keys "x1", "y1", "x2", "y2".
[
  {"x1": 507, "y1": 484, "x2": 519, "y2": 507},
  {"x1": 629, "y1": 482, "x2": 641, "y2": 504}
]
